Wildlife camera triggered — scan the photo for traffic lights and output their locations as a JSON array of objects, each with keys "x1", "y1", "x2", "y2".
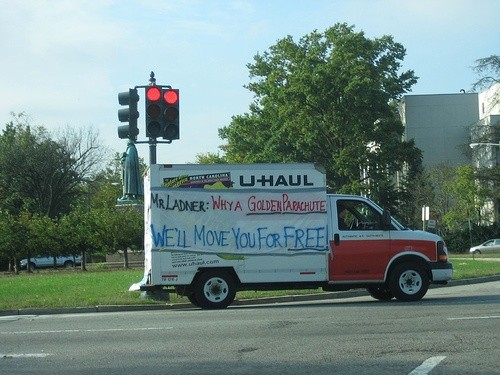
[
  {"x1": 117, "y1": 87, "x2": 139, "y2": 140},
  {"x1": 161, "y1": 88, "x2": 180, "y2": 141},
  {"x1": 145, "y1": 87, "x2": 163, "y2": 137}
]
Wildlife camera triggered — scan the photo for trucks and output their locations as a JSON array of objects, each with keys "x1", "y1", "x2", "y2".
[{"x1": 127, "y1": 163, "x2": 454, "y2": 310}]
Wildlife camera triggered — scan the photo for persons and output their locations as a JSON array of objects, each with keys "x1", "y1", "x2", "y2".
[{"x1": 337, "y1": 211, "x2": 349, "y2": 230}]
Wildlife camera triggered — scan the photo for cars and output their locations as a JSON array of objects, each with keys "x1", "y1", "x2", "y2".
[
  {"x1": 117, "y1": 245, "x2": 142, "y2": 255},
  {"x1": 20, "y1": 253, "x2": 83, "y2": 271},
  {"x1": 469, "y1": 238, "x2": 500, "y2": 254}
]
